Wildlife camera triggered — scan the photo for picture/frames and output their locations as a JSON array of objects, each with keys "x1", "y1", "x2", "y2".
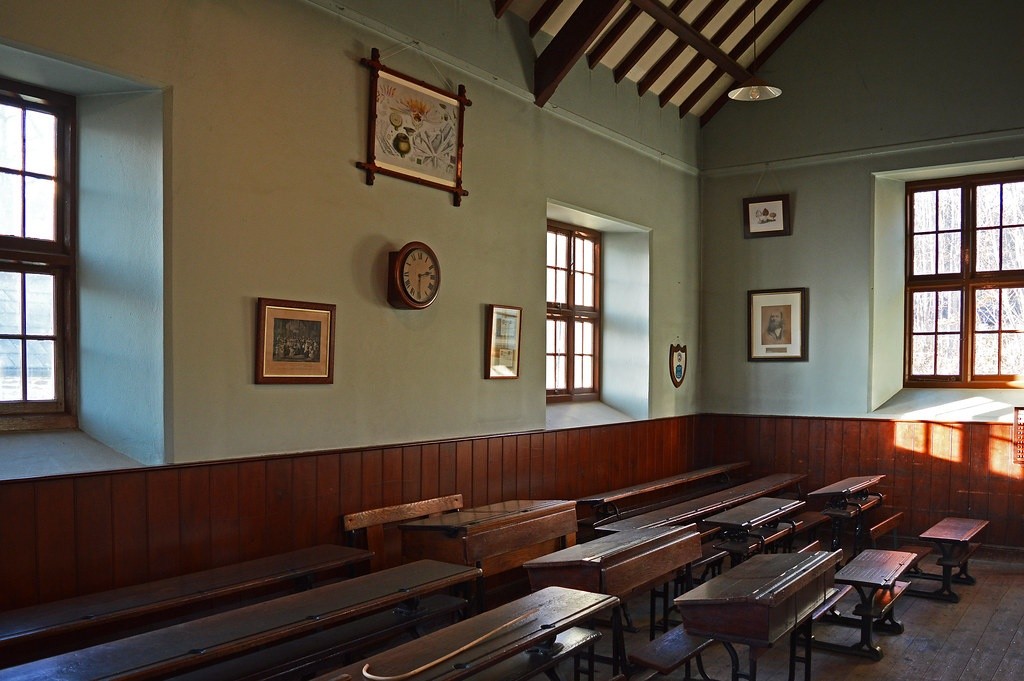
[
  {"x1": 486, "y1": 304, "x2": 522, "y2": 379},
  {"x1": 254, "y1": 298, "x2": 335, "y2": 385},
  {"x1": 747, "y1": 288, "x2": 805, "y2": 362},
  {"x1": 743, "y1": 194, "x2": 789, "y2": 239},
  {"x1": 669, "y1": 344, "x2": 687, "y2": 388},
  {"x1": 356, "y1": 47, "x2": 472, "y2": 208}
]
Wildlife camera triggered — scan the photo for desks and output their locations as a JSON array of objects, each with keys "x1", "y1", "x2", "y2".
[
  {"x1": 522, "y1": 522, "x2": 694, "y2": 681},
  {"x1": 835, "y1": 539, "x2": 920, "y2": 660},
  {"x1": 0, "y1": 559, "x2": 482, "y2": 681},
  {"x1": 0, "y1": 543, "x2": 375, "y2": 670},
  {"x1": 806, "y1": 474, "x2": 888, "y2": 571},
  {"x1": 673, "y1": 549, "x2": 843, "y2": 681},
  {"x1": 397, "y1": 499, "x2": 579, "y2": 616},
  {"x1": 702, "y1": 496, "x2": 805, "y2": 568},
  {"x1": 573, "y1": 460, "x2": 758, "y2": 529},
  {"x1": 917, "y1": 517, "x2": 990, "y2": 604},
  {"x1": 309, "y1": 586, "x2": 626, "y2": 681},
  {"x1": 593, "y1": 473, "x2": 819, "y2": 543}
]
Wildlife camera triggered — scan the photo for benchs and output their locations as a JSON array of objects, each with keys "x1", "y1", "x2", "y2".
[
  {"x1": 870, "y1": 513, "x2": 934, "y2": 597},
  {"x1": 462, "y1": 508, "x2": 576, "y2": 615},
  {"x1": 161, "y1": 591, "x2": 468, "y2": 681},
  {"x1": 600, "y1": 532, "x2": 717, "y2": 681},
  {"x1": 462, "y1": 626, "x2": 603, "y2": 681},
  {"x1": 686, "y1": 510, "x2": 831, "y2": 582},
  {"x1": 785, "y1": 540, "x2": 853, "y2": 651},
  {"x1": 343, "y1": 493, "x2": 465, "y2": 574}
]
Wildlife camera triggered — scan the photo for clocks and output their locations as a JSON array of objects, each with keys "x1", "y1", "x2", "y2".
[{"x1": 387, "y1": 241, "x2": 441, "y2": 310}]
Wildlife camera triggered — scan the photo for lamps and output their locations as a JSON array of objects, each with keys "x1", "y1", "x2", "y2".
[{"x1": 727, "y1": 0, "x2": 782, "y2": 102}]
